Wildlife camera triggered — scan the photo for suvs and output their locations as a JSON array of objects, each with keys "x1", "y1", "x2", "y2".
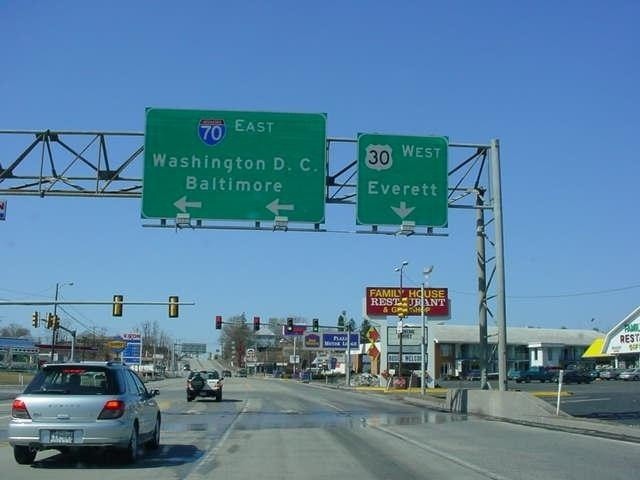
[{"x1": 186, "y1": 369, "x2": 224, "y2": 402}]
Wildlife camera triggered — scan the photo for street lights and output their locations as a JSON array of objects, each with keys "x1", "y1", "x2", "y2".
[{"x1": 394, "y1": 261, "x2": 408, "y2": 377}]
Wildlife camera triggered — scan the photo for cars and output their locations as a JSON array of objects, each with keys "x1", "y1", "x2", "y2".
[
  {"x1": 7, "y1": 360, "x2": 161, "y2": 464},
  {"x1": 507, "y1": 366, "x2": 640, "y2": 385},
  {"x1": 184, "y1": 364, "x2": 190, "y2": 371},
  {"x1": 221, "y1": 368, "x2": 248, "y2": 378}
]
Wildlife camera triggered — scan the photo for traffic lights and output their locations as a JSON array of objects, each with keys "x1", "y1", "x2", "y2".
[
  {"x1": 287, "y1": 317, "x2": 293, "y2": 332},
  {"x1": 313, "y1": 318, "x2": 319, "y2": 332},
  {"x1": 32, "y1": 311, "x2": 60, "y2": 331}
]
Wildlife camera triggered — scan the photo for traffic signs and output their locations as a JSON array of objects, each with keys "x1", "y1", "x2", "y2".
[
  {"x1": 140, "y1": 106, "x2": 328, "y2": 225},
  {"x1": 355, "y1": 132, "x2": 449, "y2": 228}
]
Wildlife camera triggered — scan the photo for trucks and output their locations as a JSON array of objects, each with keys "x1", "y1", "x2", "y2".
[{"x1": 447, "y1": 356, "x2": 488, "y2": 382}]
